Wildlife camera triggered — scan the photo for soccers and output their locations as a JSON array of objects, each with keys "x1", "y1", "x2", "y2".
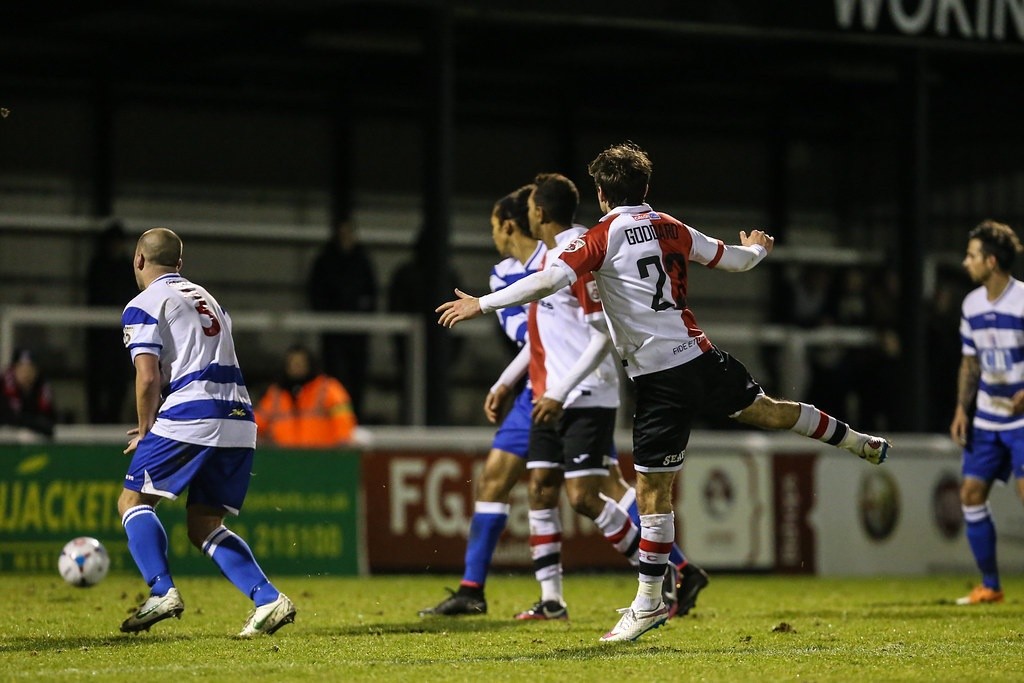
[{"x1": 57, "y1": 537, "x2": 111, "y2": 590}]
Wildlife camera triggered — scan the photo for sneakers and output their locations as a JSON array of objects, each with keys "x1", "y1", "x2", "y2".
[
  {"x1": 121, "y1": 587, "x2": 183, "y2": 632},
  {"x1": 599, "y1": 601, "x2": 668, "y2": 641},
  {"x1": 956, "y1": 584, "x2": 1003, "y2": 606},
  {"x1": 660, "y1": 561, "x2": 681, "y2": 618},
  {"x1": 853, "y1": 433, "x2": 891, "y2": 466},
  {"x1": 237, "y1": 593, "x2": 297, "y2": 637},
  {"x1": 679, "y1": 569, "x2": 708, "y2": 615},
  {"x1": 417, "y1": 586, "x2": 486, "y2": 618},
  {"x1": 513, "y1": 599, "x2": 568, "y2": 620}
]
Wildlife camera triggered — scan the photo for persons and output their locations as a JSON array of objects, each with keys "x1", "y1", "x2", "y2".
[
  {"x1": 116, "y1": 226, "x2": 296, "y2": 647},
  {"x1": 417, "y1": 140, "x2": 890, "y2": 648},
  {"x1": 761, "y1": 188, "x2": 1024, "y2": 432},
  {"x1": 83, "y1": 223, "x2": 137, "y2": 425},
  {"x1": 387, "y1": 193, "x2": 462, "y2": 425},
  {"x1": 308, "y1": 207, "x2": 381, "y2": 425},
  {"x1": 254, "y1": 342, "x2": 358, "y2": 450},
  {"x1": 0, "y1": 346, "x2": 59, "y2": 441},
  {"x1": 949, "y1": 218, "x2": 1024, "y2": 607}
]
